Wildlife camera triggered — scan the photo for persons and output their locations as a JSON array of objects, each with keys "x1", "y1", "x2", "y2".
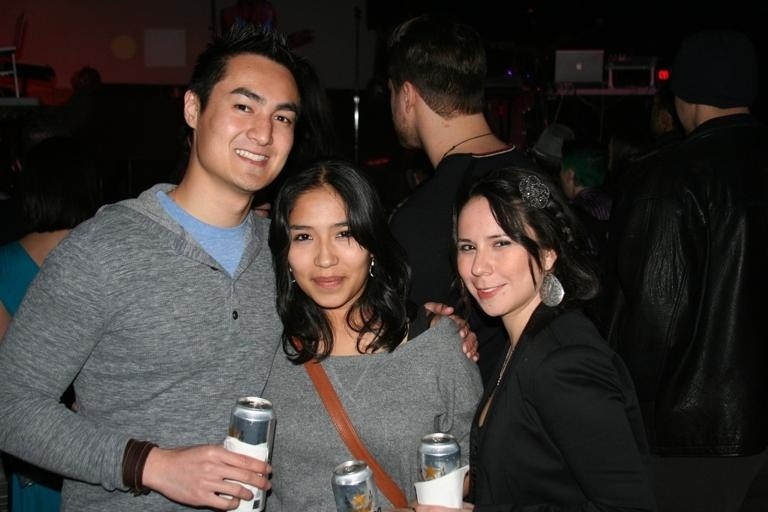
[
  {"x1": 0, "y1": 40, "x2": 480, "y2": 511},
  {"x1": 374, "y1": 12, "x2": 569, "y2": 320},
  {"x1": 1, "y1": 139, "x2": 155, "y2": 512},
  {"x1": 632, "y1": 33, "x2": 768, "y2": 511},
  {"x1": 452, "y1": 165, "x2": 653, "y2": 509},
  {"x1": 649, "y1": 83, "x2": 689, "y2": 142},
  {"x1": 559, "y1": 144, "x2": 613, "y2": 245},
  {"x1": 254, "y1": 162, "x2": 484, "y2": 510}
]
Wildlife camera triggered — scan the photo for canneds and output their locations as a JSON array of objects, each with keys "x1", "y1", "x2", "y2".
[
  {"x1": 218, "y1": 396, "x2": 277, "y2": 512},
  {"x1": 417, "y1": 433, "x2": 461, "y2": 481},
  {"x1": 331, "y1": 461, "x2": 382, "y2": 512}
]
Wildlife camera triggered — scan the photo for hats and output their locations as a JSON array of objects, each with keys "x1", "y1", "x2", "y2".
[{"x1": 669, "y1": 29, "x2": 759, "y2": 110}]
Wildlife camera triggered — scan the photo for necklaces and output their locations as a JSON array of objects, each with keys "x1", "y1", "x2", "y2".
[
  {"x1": 495, "y1": 346, "x2": 514, "y2": 386},
  {"x1": 443, "y1": 130, "x2": 492, "y2": 157}
]
